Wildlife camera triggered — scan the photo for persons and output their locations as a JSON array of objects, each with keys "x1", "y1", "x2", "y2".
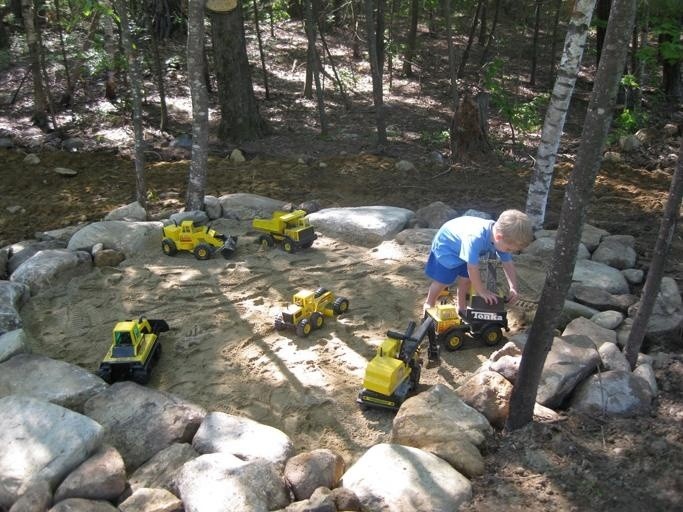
[{"x1": 421, "y1": 207, "x2": 534, "y2": 317}]
